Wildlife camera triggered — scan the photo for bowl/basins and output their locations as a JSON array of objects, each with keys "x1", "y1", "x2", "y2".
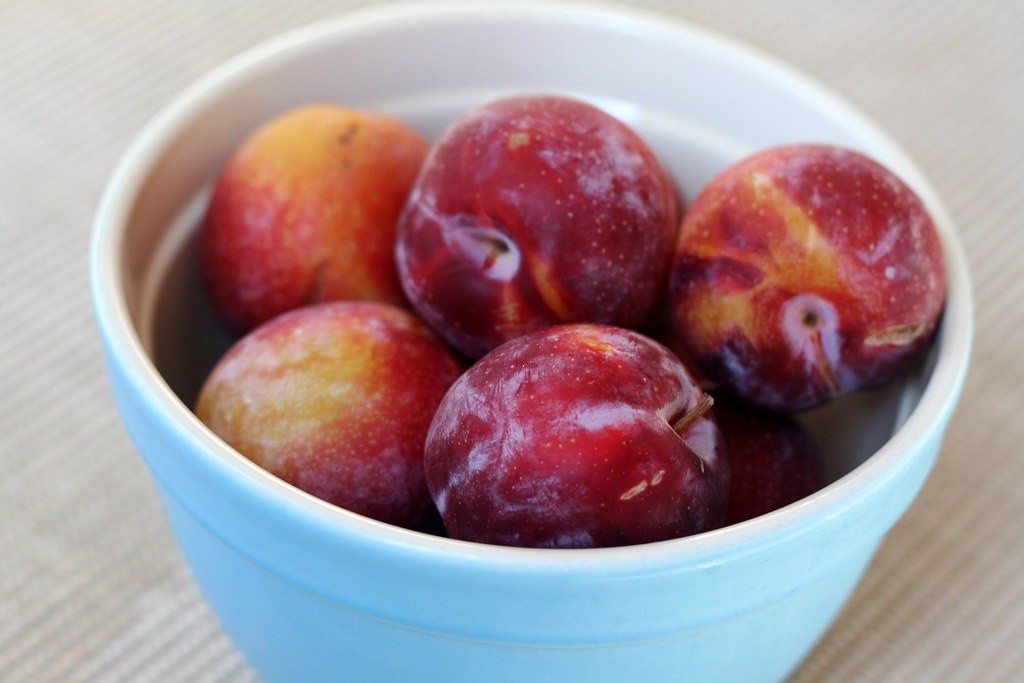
[{"x1": 84, "y1": 5, "x2": 974, "y2": 683}]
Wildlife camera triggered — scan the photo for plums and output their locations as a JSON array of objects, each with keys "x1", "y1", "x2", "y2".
[{"x1": 193, "y1": 93, "x2": 947, "y2": 550}]
